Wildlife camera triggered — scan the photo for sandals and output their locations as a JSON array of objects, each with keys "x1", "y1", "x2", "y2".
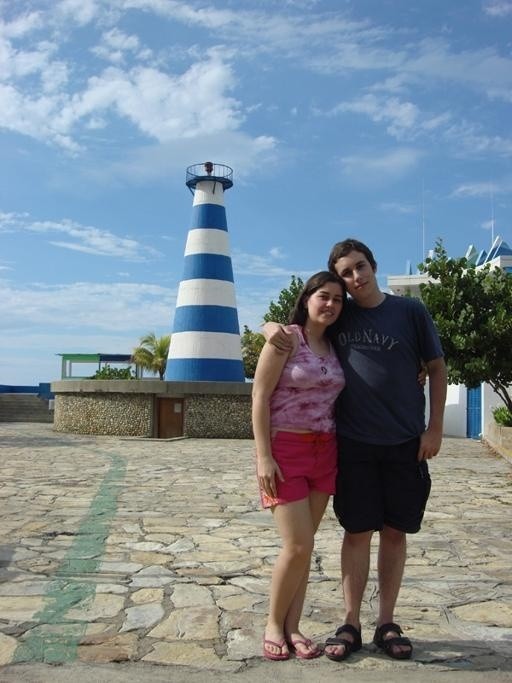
[
  {"x1": 324, "y1": 624, "x2": 362, "y2": 661},
  {"x1": 373, "y1": 622, "x2": 413, "y2": 660}
]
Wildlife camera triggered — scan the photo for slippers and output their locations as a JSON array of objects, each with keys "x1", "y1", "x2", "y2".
[
  {"x1": 286, "y1": 638, "x2": 321, "y2": 659},
  {"x1": 263, "y1": 631, "x2": 290, "y2": 660}
]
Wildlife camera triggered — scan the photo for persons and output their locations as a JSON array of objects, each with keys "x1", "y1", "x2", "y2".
[
  {"x1": 251, "y1": 271, "x2": 428, "y2": 661},
  {"x1": 262, "y1": 237, "x2": 448, "y2": 659}
]
